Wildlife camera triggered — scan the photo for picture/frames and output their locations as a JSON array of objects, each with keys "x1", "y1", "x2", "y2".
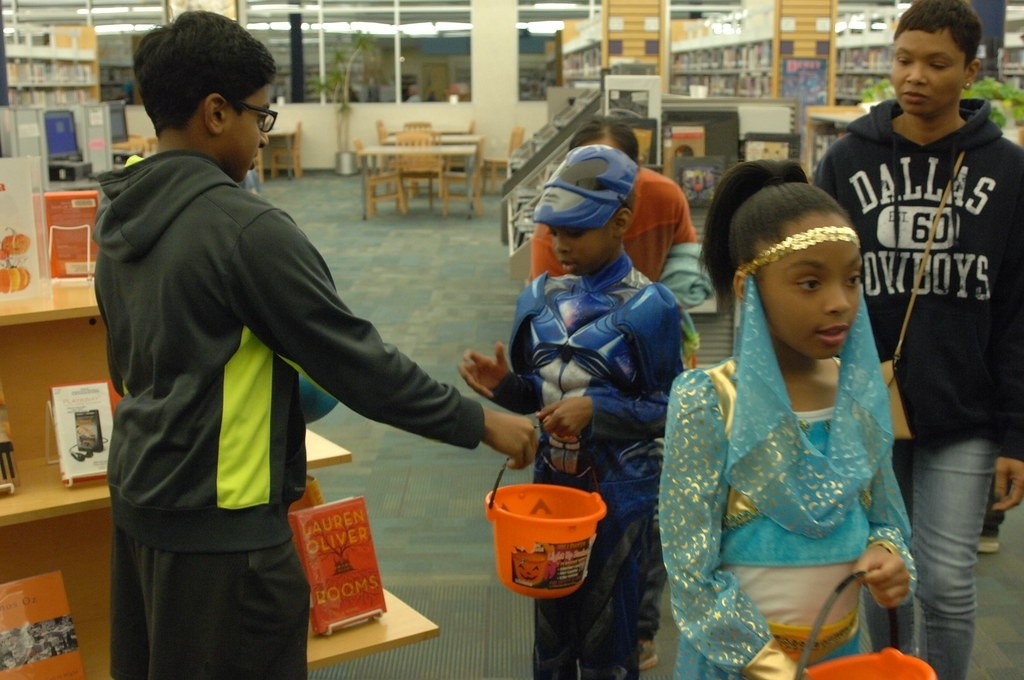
[{"x1": 660, "y1": 121, "x2": 726, "y2": 209}]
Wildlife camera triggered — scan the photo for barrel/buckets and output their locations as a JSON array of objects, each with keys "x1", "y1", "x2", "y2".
[
  {"x1": 794, "y1": 569, "x2": 938, "y2": 680},
  {"x1": 485, "y1": 422, "x2": 607, "y2": 599}
]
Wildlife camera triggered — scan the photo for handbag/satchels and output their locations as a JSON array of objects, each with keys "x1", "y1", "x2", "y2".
[{"x1": 877, "y1": 357, "x2": 913, "y2": 441}]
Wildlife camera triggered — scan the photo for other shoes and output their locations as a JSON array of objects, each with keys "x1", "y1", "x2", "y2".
[{"x1": 975, "y1": 535, "x2": 1001, "y2": 554}]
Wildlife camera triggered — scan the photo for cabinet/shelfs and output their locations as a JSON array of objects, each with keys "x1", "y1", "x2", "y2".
[
  {"x1": 500, "y1": 90, "x2": 657, "y2": 290},
  {"x1": 99, "y1": 61, "x2": 133, "y2": 102},
  {"x1": 4, "y1": 25, "x2": 99, "y2": 106},
  {"x1": 543, "y1": 0, "x2": 1024, "y2": 184},
  {"x1": 0, "y1": 279, "x2": 441, "y2": 680}
]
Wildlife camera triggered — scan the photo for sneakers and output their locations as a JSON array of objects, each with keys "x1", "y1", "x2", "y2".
[{"x1": 637, "y1": 639, "x2": 657, "y2": 670}]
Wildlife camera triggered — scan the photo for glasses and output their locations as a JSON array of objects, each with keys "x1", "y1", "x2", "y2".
[{"x1": 225, "y1": 96, "x2": 277, "y2": 132}]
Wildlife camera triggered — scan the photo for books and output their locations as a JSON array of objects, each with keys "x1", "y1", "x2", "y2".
[
  {"x1": 605, "y1": 39, "x2": 1024, "y2": 205},
  {"x1": 0, "y1": 58, "x2": 386, "y2": 680}
]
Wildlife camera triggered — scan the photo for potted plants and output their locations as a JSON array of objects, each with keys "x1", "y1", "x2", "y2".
[{"x1": 306, "y1": 29, "x2": 378, "y2": 176}]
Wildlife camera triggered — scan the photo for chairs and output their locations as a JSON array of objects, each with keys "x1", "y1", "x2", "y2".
[
  {"x1": 352, "y1": 118, "x2": 525, "y2": 219},
  {"x1": 271, "y1": 122, "x2": 304, "y2": 179}
]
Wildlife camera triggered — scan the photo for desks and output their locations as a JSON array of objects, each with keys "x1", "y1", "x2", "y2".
[
  {"x1": 357, "y1": 144, "x2": 477, "y2": 219},
  {"x1": 381, "y1": 134, "x2": 485, "y2": 147},
  {"x1": 265, "y1": 127, "x2": 296, "y2": 179}
]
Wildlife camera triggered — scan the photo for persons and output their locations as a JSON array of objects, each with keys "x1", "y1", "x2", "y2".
[
  {"x1": 977, "y1": 474, "x2": 1012, "y2": 552},
  {"x1": 814, "y1": 0, "x2": 1024, "y2": 680},
  {"x1": 90, "y1": 11, "x2": 559, "y2": 680},
  {"x1": 458, "y1": 116, "x2": 712, "y2": 679},
  {"x1": 658, "y1": 158, "x2": 921, "y2": 680}
]
